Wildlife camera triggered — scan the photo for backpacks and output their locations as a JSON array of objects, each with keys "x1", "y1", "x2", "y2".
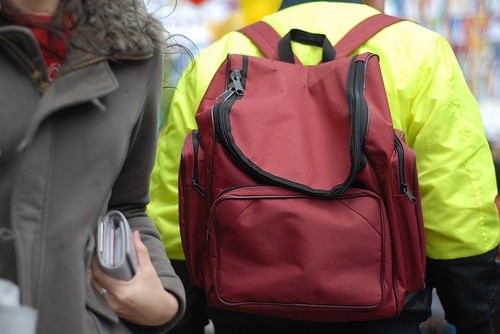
[{"x1": 175, "y1": 11, "x2": 427, "y2": 325}]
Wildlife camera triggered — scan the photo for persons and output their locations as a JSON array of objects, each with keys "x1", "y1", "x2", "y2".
[
  {"x1": 0, "y1": 0, "x2": 187, "y2": 334},
  {"x1": 143, "y1": 0, "x2": 500, "y2": 334}
]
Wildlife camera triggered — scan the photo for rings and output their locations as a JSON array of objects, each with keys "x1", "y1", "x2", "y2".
[{"x1": 98, "y1": 287, "x2": 108, "y2": 297}]
